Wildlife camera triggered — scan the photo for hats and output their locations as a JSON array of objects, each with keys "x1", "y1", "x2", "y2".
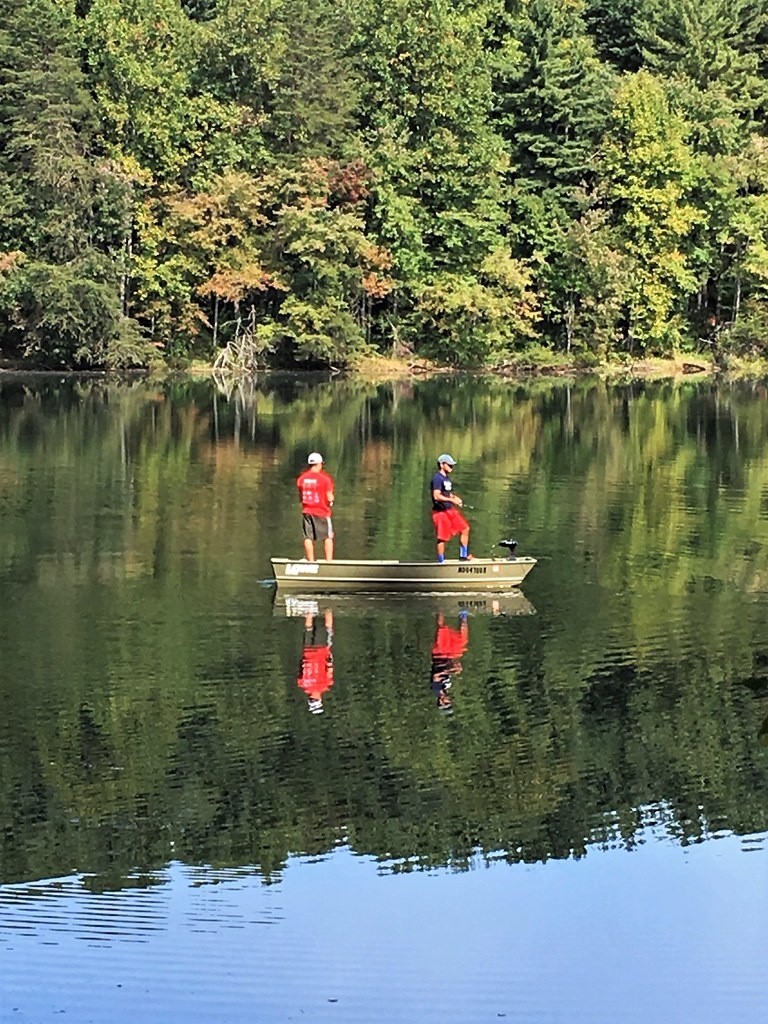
[
  {"x1": 307, "y1": 699, "x2": 324, "y2": 714},
  {"x1": 308, "y1": 453, "x2": 323, "y2": 464},
  {"x1": 438, "y1": 454, "x2": 457, "y2": 465},
  {"x1": 438, "y1": 704, "x2": 453, "y2": 715}
]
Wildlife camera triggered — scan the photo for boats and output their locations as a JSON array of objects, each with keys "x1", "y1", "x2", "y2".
[
  {"x1": 269, "y1": 539, "x2": 538, "y2": 589},
  {"x1": 270, "y1": 586, "x2": 540, "y2": 617}
]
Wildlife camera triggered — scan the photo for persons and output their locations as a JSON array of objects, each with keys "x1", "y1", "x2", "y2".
[
  {"x1": 431, "y1": 607, "x2": 469, "y2": 710},
  {"x1": 296, "y1": 608, "x2": 335, "y2": 716},
  {"x1": 297, "y1": 453, "x2": 335, "y2": 562},
  {"x1": 430, "y1": 454, "x2": 471, "y2": 563}
]
